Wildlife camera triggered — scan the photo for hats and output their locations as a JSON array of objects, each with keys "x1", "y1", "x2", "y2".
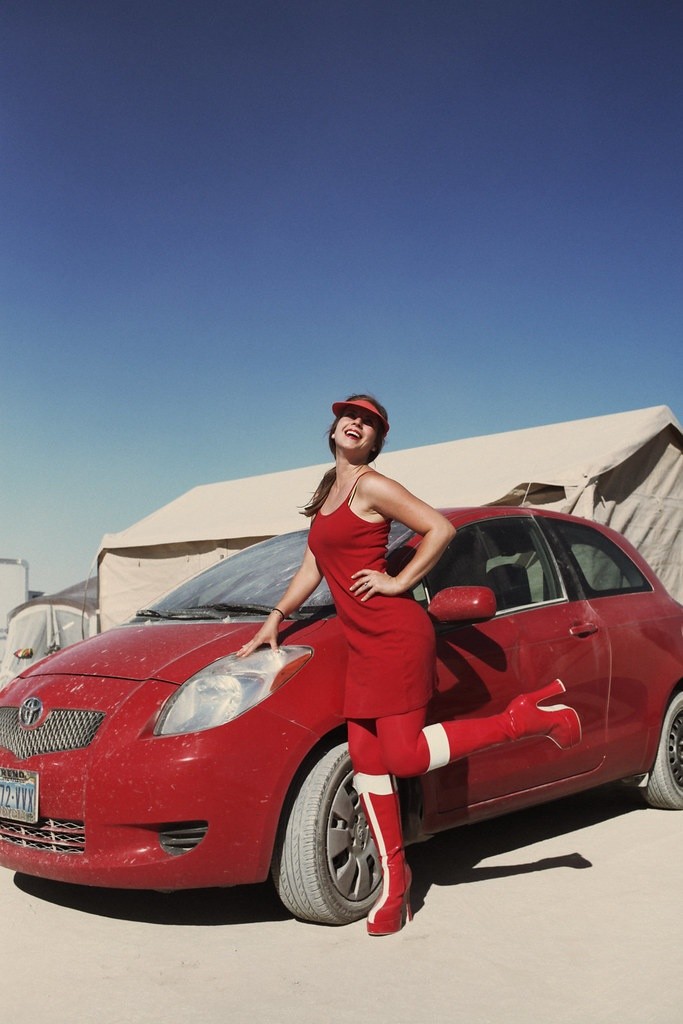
[{"x1": 332, "y1": 396, "x2": 389, "y2": 436}]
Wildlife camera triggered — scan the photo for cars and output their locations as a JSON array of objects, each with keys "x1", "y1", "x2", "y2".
[{"x1": 1, "y1": 508, "x2": 683, "y2": 932}]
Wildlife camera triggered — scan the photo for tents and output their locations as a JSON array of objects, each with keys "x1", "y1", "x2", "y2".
[
  {"x1": 0, "y1": 575, "x2": 97, "y2": 690},
  {"x1": 95, "y1": 403, "x2": 683, "y2": 635}
]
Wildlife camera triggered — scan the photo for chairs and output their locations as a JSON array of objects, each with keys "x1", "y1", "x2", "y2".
[{"x1": 478, "y1": 563, "x2": 531, "y2": 612}]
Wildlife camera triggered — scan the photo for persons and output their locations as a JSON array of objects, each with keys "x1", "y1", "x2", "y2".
[{"x1": 233, "y1": 394, "x2": 582, "y2": 936}]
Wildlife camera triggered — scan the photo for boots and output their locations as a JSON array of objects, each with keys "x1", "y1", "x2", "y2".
[
  {"x1": 418, "y1": 677, "x2": 583, "y2": 775},
  {"x1": 350, "y1": 771, "x2": 413, "y2": 936}
]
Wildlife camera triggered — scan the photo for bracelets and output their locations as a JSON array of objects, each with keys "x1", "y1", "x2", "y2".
[{"x1": 272, "y1": 608, "x2": 284, "y2": 620}]
